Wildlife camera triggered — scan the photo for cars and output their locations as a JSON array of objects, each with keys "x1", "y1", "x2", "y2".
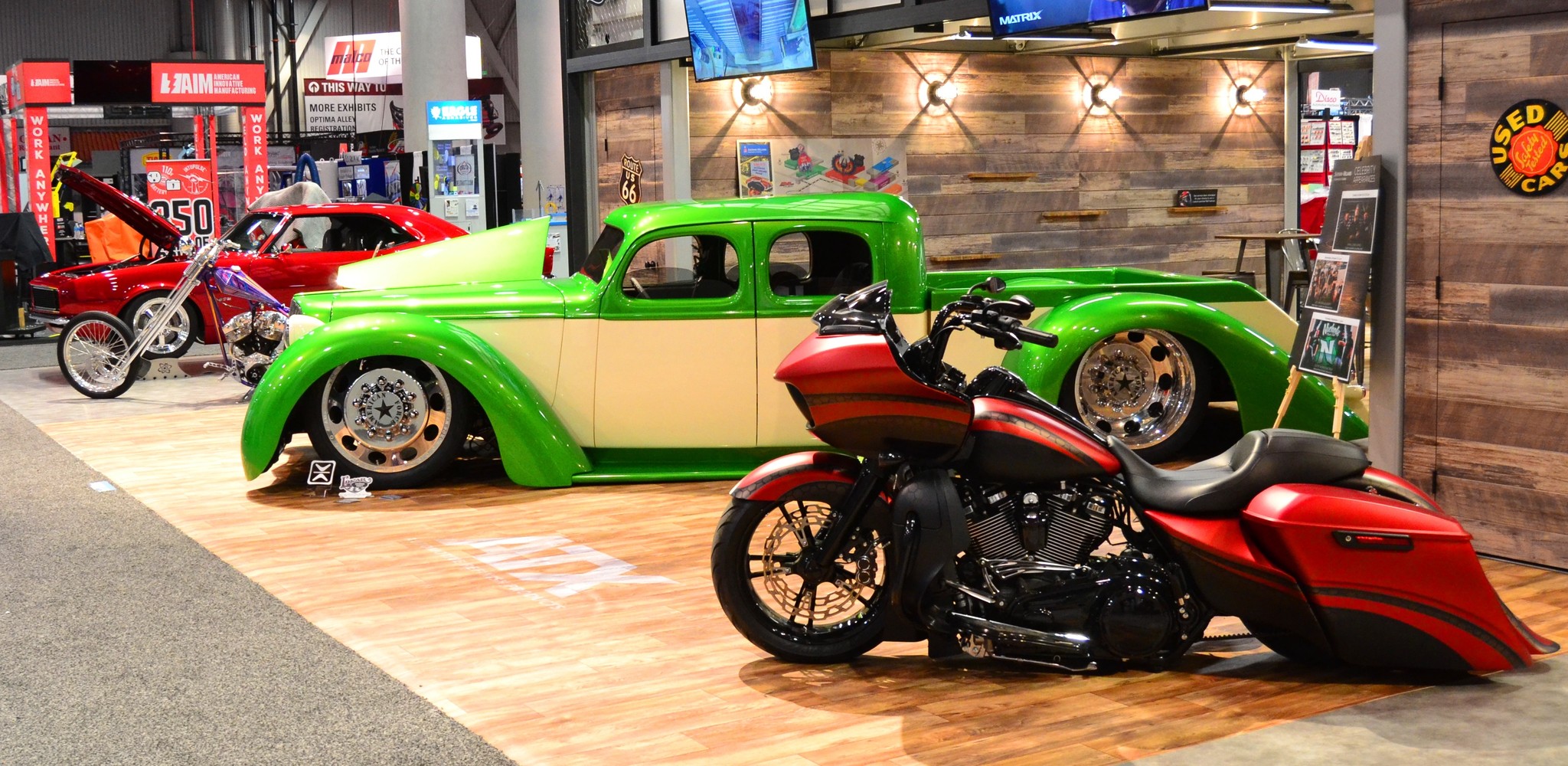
[
  {"x1": 26, "y1": 162, "x2": 555, "y2": 374},
  {"x1": 236, "y1": 193, "x2": 1376, "y2": 523}
]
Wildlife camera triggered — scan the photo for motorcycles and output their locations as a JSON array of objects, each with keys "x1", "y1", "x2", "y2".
[
  {"x1": 710, "y1": 271, "x2": 1564, "y2": 686},
  {"x1": 54, "y1": 239, "x2": 297, "y2": 408}
]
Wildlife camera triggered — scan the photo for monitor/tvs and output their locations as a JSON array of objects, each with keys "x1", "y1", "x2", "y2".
[
  {"x1": 683, "y1": 0, "x2": 818, "y2": 84},
  {"x1": 986, "y1": 0, "x2": 1208, "y2": 40}
]
